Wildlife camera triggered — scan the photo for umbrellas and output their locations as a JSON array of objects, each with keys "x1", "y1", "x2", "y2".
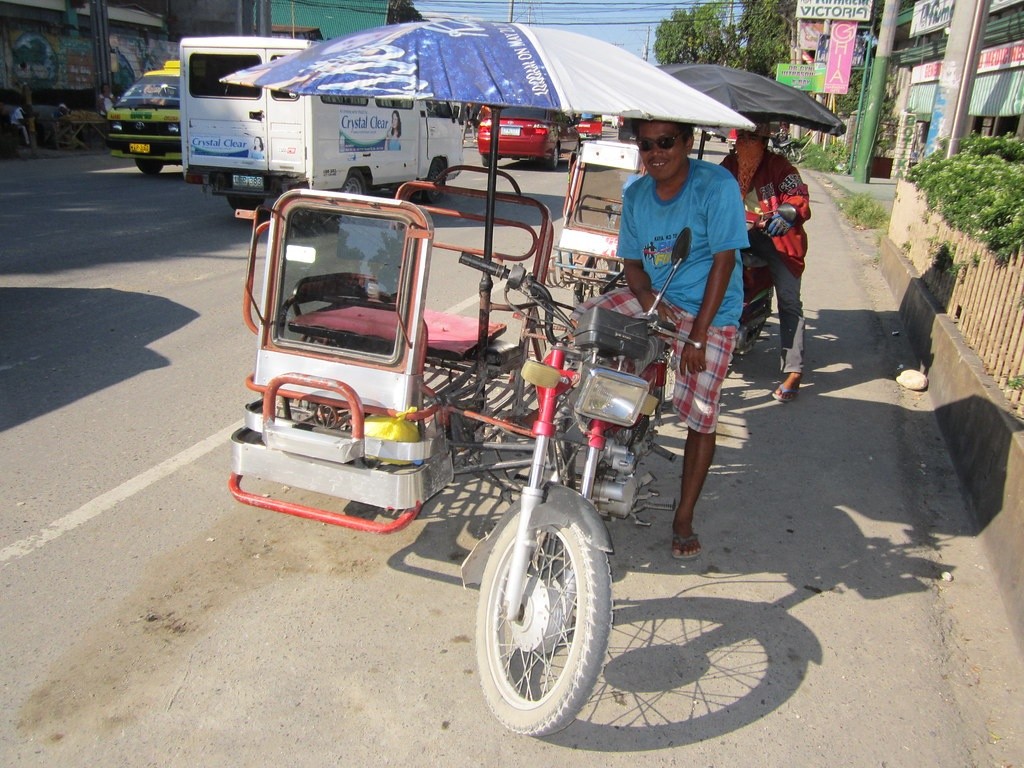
[
  {"x1": 218, "y1": 19, "x2": 756, "y2": 399},
  {"x1": 658, "y1": 65, "x2": 846, "y2": 161}
]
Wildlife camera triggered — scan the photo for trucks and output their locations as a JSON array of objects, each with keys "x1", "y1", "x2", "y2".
[{"x1": 178, "y1": 35, "x2": 464, "y2": 216}]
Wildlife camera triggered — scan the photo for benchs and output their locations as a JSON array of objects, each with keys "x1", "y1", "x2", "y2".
[{"x1": 288, "y1": 164, "x2": 555, "y2": 361}]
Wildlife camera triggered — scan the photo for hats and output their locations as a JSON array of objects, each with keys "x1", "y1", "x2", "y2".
[{"x1": 736, "y1": 122, "x2": 775, "y2": 137}]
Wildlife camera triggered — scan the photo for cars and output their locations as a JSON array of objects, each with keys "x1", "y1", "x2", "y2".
[
  {"x1": 727, "y1": 128, "x2": 737, "y2": 149},
  {"x1": 618, "y1": 116, "x2": 638, "y2": 143},
  {"x1": 611, "y1": 116, "x2": 619, "y2": 130},
  {"x1": 477, "y1": 108, "x2": 582, "y2": 171},
  {"x1": 573, "y1": 115, "x2": 602, "y2": 140}
]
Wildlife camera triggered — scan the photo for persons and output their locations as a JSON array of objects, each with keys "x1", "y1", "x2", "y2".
[
  {"x1": 12, "y1": 107, "x2": 31, "y2": 145},
  {"x1": 463, "y1": 102, "x2": 477, "y2": 139},
  {"x1": 617, "y1": 119, "x2": 750, "y2": 558},
  {"x1": 719, "y1": 118, "x2": 811, "y2": 402},
  {"x1": 385, "y1": 110, "x2": 402, "y2": 150},
  {"x1": 97, "y1": 84, "x2": 115, "y2": 115}
]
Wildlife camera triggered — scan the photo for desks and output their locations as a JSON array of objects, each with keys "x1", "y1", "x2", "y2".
[{"x1": 57, "y1": 118, "x2": 106, "y2": 150}]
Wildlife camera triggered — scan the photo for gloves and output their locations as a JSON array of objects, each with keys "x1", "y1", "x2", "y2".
[{"x1": 767, "y1": 214, "x2": 796, "y2": 237}]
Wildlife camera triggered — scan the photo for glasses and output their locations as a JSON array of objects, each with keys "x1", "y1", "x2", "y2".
[{"x1": 636, "y1": 130, "x2": 684, "y2": 152}]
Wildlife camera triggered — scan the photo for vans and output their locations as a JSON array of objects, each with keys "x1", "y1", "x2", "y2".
[{"x1": 103, "y1": 60, "x2": 183, "y2": 174}]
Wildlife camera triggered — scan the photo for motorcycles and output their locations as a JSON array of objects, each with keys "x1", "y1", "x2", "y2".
[
  {"x1": 227, "y1": 164, "x2": 704, "y2": 737},
  {"x1": 545, "y1": 144, "x2": 797, "y2": 358},
  {"x1": 767, "y1": 129, "x2": 802, "y2": 165}
]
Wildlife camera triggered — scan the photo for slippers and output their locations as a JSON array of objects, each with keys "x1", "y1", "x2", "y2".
[
  {"x1": 772, "y1": 383, "x2": 799, "y2": 402},
  {"x1": 671, "y1": 534, "x2": 703, "y2": 559}
]
